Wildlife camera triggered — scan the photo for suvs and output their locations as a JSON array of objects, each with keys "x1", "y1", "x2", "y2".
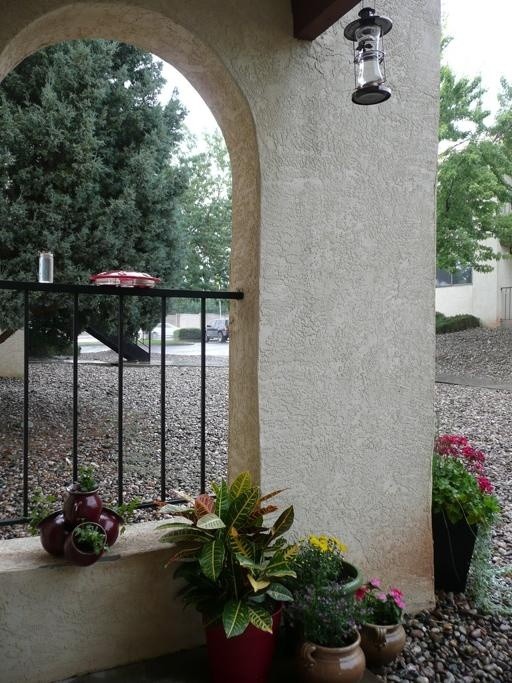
[{"x1": 205, "y1": 317, "x2": 230, "y2": 344}]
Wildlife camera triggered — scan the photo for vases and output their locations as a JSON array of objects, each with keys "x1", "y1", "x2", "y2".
[
  {"x1": 359, "y1": 617, "x2": 406, "y2": 666},
  {"x1": 431, "y1": 516, "x2": 478, "y2": 594},
  {"x1": 286, "y1": 559, "x2": 363, "y2": 605}
]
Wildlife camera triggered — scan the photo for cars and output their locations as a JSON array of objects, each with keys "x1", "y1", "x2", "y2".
[{"x1": 152, "y1": 323, "x2": 180, "y2": 339}]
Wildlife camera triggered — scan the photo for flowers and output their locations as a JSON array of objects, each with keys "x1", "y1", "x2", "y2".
[
  {"x1": 293, "y1": 532, "x2": 348, "y2": 587},
  {"x1": 432, "y1": 433, "x2": 503, "y2": 526},
  {"x1": 353, "y1": 577, "x2": 406, "y2": 624}
]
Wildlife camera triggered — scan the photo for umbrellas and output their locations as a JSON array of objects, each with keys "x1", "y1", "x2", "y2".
[{"x1": 88, "y1": 268, "x2": 164, "y2": 287}]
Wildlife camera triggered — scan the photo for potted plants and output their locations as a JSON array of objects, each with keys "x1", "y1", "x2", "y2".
[
  {"x1": 27, "y1": 467, "x2": 141, "y2": 566},
  {"x1": 279, "y1": 580, "x2": 364, "y2": 683},
  {"x1": 153, "y1": 469, "x2": 307, "y2": 683}
]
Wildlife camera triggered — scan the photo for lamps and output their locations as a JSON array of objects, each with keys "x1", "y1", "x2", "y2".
[{"x1": 344, "y1": 0, "x2": 392, "y2": 105}]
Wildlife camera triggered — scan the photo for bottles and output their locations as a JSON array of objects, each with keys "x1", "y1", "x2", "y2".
[{"x1": 39, "y1": 252, "x2": 54, "y2": 283}]
